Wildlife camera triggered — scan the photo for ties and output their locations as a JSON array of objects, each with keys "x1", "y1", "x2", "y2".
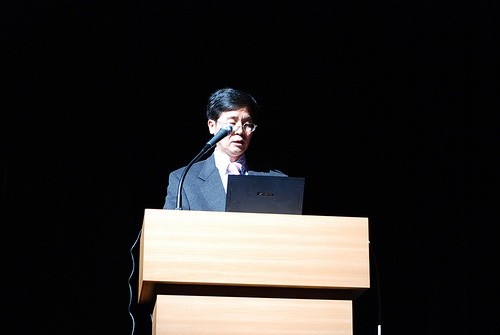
[{"x1": 226, "y1": 164, "x2": 241, "y2": 175}]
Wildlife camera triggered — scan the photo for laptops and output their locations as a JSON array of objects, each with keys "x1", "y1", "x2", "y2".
[{"x1": 225, "y1": 174, "x2": 305, "y2": 215}]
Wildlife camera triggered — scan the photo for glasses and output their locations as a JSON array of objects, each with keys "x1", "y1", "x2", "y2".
[{"x1": 216, "y1": 122, "x2": 258, "y2": 133}]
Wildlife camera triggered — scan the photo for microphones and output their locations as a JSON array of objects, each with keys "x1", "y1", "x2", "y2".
[{"x1": 175, "y1": 124, "x2": 233, "y2": 210}]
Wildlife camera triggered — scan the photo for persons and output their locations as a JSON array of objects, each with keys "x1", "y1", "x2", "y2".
[{"x1": 162, "y1": 87, "x2": 288, "y2": 211}]
